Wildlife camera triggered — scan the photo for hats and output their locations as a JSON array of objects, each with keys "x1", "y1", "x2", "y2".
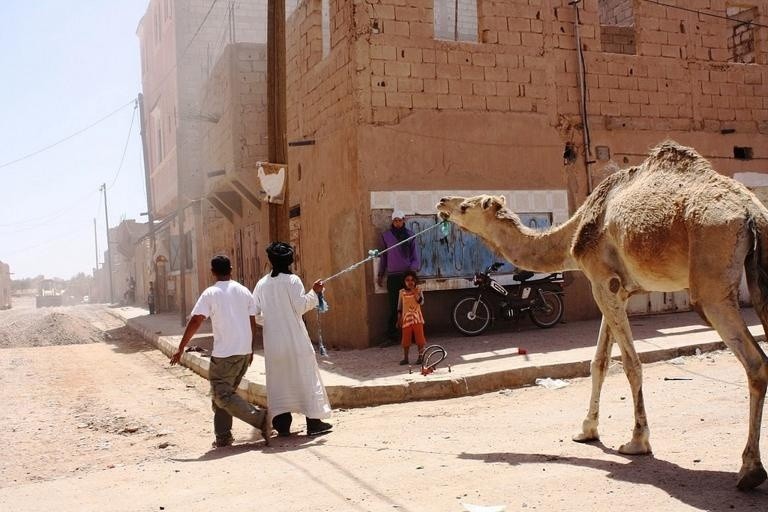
[
  {"x1": 391, "y1": 210, "x2": 406, "y2": 222},
  {"x1": 267, "y1": 243, "x2": 295, "y2": 266}
]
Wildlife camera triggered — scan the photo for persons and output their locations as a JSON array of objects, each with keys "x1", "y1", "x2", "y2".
[
  {"x1": 147, "y1": 287, "x2": 154, "y2": 315},
  {"x1": 169, "y1": 255, "x2": 271, "y2": 449},
  {"x1": 127, "y1": 277, "x2": 135, "y2": 304},
  {"x1": 395, "y1": 273, "x2": 425, "y2": 365},
  {"x1": 252, "y1": 241, "x2": 332, "y2": 437},
  {"x1": 147, "y1": 281, "x2": 152, "y2": 295},
  {"x1": 377, "y1": 210, "x2": 419, "y2": 348}
]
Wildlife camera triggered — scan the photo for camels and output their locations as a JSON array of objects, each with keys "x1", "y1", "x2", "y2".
[{"x1": 436, "y1": 138, "x2": 768, "y2": 491}]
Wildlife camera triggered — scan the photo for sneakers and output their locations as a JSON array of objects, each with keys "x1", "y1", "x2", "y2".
[
  {"x1": 398, "y1": 355, "x2": 424, "y2": 366},
  {"x1": 259, "y1": 411, "x2": 270, "y2": 445},
  {"x1": 308, "y1": 424, "x2": 333, "y2": 435}
]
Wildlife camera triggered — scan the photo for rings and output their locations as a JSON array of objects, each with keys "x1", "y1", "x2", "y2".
[
  {"x1": 171, "y1": 360, "x2": 173, "y2": 361},
  {"x1": 175, "y1": 360, "x2": 176, "y2": 361}
]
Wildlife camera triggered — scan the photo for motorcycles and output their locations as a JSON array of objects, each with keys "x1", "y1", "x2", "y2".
[{"x1": 451, "y1": 261, "x2": 568, "y2": 336}]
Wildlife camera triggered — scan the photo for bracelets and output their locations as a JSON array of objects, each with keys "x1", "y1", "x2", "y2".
[{"x1": 415, "y1": 294, "x2": 418, "y2": 298}]
